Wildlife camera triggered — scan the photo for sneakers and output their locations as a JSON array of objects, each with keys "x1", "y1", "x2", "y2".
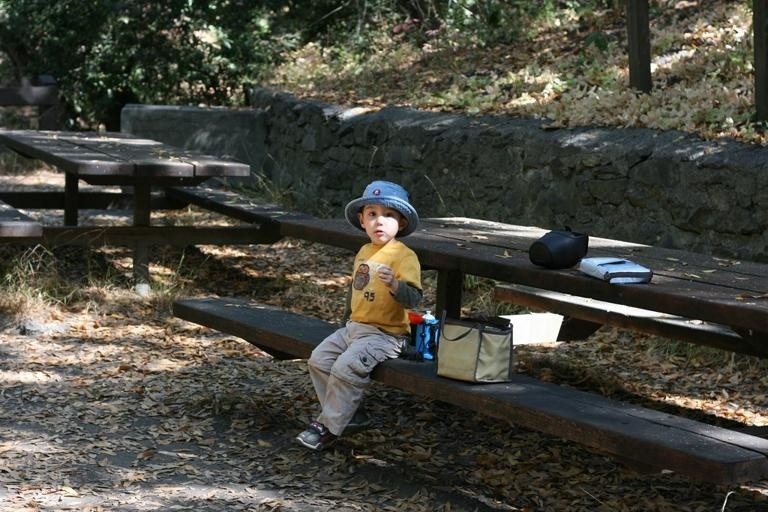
[
  {"x1": 295, "y1": 420, "x2": 339, "y2": 452},
  {"x1": 342, "y1": 410, "x2": 375, "y2": 433}
]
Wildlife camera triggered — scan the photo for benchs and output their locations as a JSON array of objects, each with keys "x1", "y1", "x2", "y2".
[
  {"x1": 494, "y1": 284, "x2": 768, "y2": 359},
  {"x1": 147, "y1": 187, "x2": 305, "y2": 245},
  {"x1": 174, "y1": 298, "x2": 768, "y2": 486},
  {"x1": 2, "y1": 185, "x2": 65, "y2": 246}
]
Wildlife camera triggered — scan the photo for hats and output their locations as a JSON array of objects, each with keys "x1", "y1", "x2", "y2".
[{"x1": 343, "y1": 179, "x2": 420, "y2": 238}]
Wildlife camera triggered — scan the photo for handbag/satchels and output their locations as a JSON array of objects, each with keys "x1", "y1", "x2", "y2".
[{"x1": 436, "y1": 309, "x2": 514, "y2": 384}]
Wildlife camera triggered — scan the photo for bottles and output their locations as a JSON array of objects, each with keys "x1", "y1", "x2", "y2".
[{"x1": 408, "y1": 310, "x2": 439, "y2": 360}]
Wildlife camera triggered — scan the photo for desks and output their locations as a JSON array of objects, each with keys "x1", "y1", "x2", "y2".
[
  {"x1": 277, "y1": 218, "x2": 767, "y2": 320},
  {"x1": 2, "y1": 131, "x2": 250, "y2": 287}
]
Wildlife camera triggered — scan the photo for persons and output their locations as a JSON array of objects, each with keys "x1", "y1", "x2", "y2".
[{"x1": 294, "y1": 179, "x2": 425, "y2": 451}]
